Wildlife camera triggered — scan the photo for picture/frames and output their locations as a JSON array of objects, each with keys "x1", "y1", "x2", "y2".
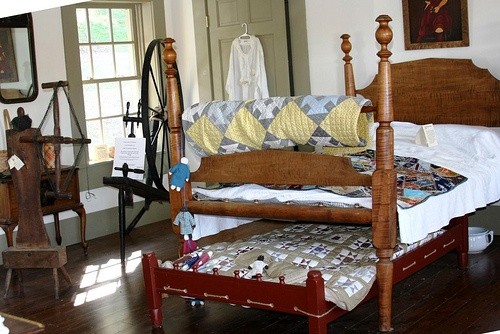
[
  {"x1": 402, "y1": 0, "x2": 469, "y2": 50},
  {"x1": 0, "y1": 28, "x2": 19, "y2": 83}
]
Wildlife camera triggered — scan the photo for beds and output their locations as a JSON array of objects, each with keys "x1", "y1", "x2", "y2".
[{"x1": 141, "y1": 15, "x2": 500, "y2": 333}]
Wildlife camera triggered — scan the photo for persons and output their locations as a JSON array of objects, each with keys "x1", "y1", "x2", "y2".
[{"x1": 415, "y1": 0, "x2": 453, "y2": 42}]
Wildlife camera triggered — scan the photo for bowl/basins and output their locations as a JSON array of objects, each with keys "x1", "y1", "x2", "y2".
[{"x1": 469, "y1": 227, "x2": 493, "y2": 254}]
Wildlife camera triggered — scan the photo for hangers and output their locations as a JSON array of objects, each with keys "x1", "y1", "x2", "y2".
[{"x1": 232, "y1": 23, "x2": 260, "y2": 44}]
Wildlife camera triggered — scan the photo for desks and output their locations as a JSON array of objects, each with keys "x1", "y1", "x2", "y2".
[{"x1": 0, "y1": 165, "x2": 89, "y2": 256}]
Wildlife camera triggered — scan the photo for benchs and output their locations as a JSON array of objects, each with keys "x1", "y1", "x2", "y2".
[{"x1": 2, "y1": 245, "x2": 74, "y2": 300}]
